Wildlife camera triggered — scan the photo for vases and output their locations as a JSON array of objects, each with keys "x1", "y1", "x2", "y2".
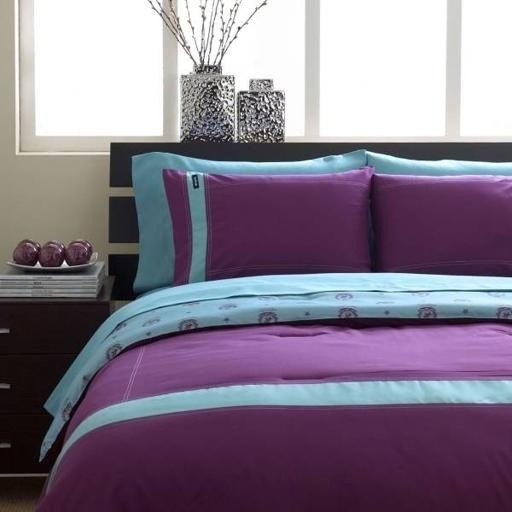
[{"x1": 180, "y1": 65, "x2": 236, "y2": 143}]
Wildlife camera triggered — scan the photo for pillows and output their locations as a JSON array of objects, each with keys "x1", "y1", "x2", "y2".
[
  {"x1": 368, "y1": 174, "x2": 512, "y2": 277},
  {"x1": 159, "y1": 165, "x2": 375, "y2": 284},
  {"x1": 365, "y1": 151, "x2": 512, "y2": 176},
  {"x1": 130, "y1": 149, "x2": 374, "y2": 292}
]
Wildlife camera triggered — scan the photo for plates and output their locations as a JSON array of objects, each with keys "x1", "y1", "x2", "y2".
[{"x1": 6, "y1": 252, "x2": 99, "y2": 271}]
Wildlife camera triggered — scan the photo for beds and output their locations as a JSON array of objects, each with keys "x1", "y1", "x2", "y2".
[{"x1": 33, "y1": 143, "x2": 512, "y2": 512}]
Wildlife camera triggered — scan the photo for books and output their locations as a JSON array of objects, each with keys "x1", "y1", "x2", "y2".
[{"x1": 0, "y1": 260, "x2": 107, "y2": 299}]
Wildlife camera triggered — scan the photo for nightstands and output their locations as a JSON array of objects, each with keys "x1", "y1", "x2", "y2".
[{"x1": 0, "y1": 275, "x2": 117, "y2": 488}]
